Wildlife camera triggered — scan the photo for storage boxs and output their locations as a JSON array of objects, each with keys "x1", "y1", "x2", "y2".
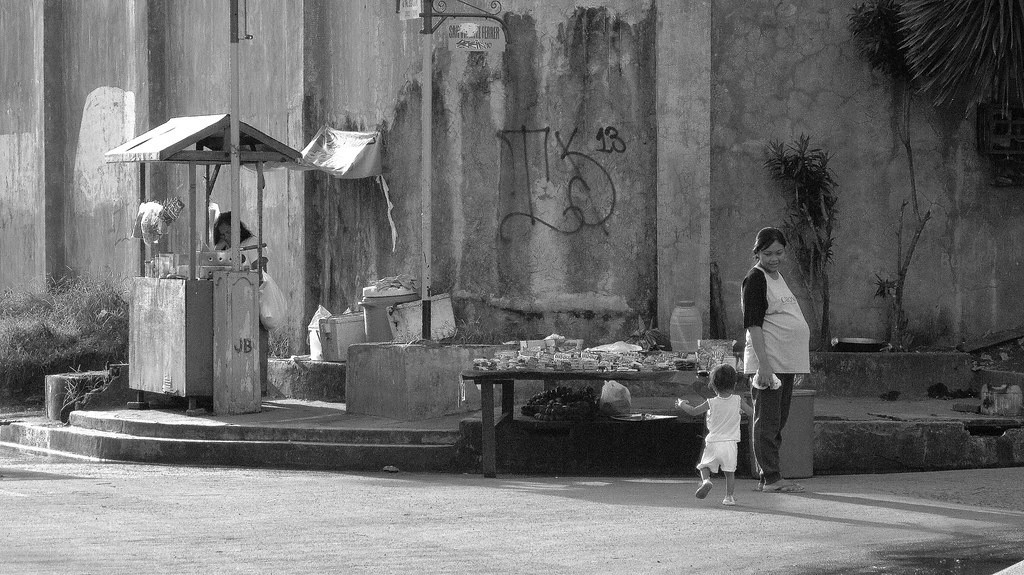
[
  {"x1": 387, "y1": 293, "x2": 457, "y2": 343},
  {"x1": 519, "y1": 340, "x2": 585, "y2": 352},
  {"x1": 319, "y1": 314, "x2": 364, "y2": 363}
]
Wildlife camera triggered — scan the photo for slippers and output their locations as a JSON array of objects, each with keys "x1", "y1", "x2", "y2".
[
  {"x1": 762, "y1": 482, "x2": 806, "y2": 494},
  {"x1": 757, "y1": 483, "x2": 764, "y2": 491}
]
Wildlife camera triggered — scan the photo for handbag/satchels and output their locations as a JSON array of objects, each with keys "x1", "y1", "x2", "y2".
[
  {"x1": 599, "y1": 380, "x2": 631, "y2": 417},
  {"x1": 259, "y1": 266, "x2": 287, "y2": 332}
]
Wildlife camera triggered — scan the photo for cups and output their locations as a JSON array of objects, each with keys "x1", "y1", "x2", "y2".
[{"x1": 145, "y1": 251, "x2": 173, "y2": 277}]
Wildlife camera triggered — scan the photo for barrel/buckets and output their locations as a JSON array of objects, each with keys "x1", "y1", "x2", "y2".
[
  {"x1": 358, "y1": 286, "x2": 418, "y2": 343},
  {"x1": 670, "y1": 301, "x2": 702, "y2": 353}
]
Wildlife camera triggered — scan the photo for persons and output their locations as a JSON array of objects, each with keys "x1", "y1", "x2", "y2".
[
  {"x1": 740, "y1": 226, "x2": 812, "y2": 491},
  {"x1": 674, "y1": 363, "x2": 753, "y2": 505},
  {"x1": 212, "y1": 211, "x2": 269, "y2": 286}
]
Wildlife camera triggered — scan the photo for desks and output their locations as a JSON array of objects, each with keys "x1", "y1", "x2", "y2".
[{"x1": 461, "y1": 369, "x2": 748, "y2": 477}]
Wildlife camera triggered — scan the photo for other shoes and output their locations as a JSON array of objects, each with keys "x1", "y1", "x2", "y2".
[
  {"x1": 695, "y1": 479, "x2": 714, "y2": 499},
  {"x1": 723, "y1": 496, "x2": 736, "y2": 506}
]
